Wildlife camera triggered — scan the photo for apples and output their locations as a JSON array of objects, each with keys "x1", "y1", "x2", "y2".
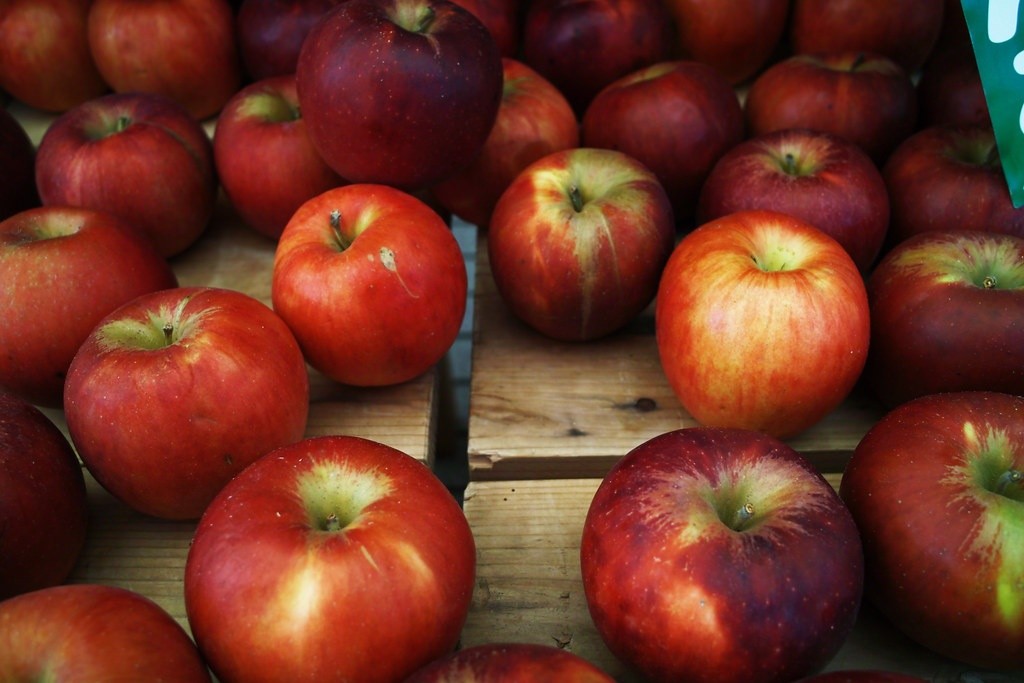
[{"x1": 0, "y1": 0, "x2": 1024, "y2": 683}]
